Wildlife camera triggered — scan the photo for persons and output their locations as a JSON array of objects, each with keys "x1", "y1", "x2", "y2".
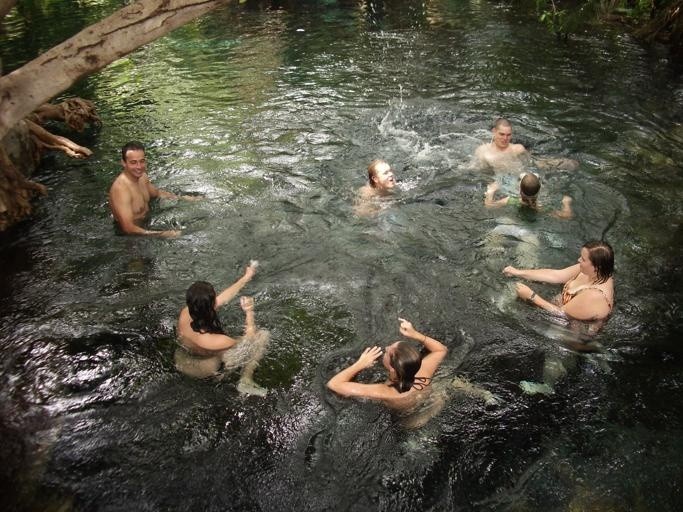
[
  {"x1": 326, "y1": 316, "x2": 501, "y2": 427},
  {"x1": 474, "y1": 116, "x2": 581, "y2": 179},
  {"x1": 108, "y1": 141, "x2": 204, "y2": 240},
  {"x1": 500, "y1": 235, "x2": 616, "y2": 401},
  {"x1": 347, "y1": 159, "x2": 411, "y2": 222},
  {"x1": 483, "y1": 172, "x2": 572, "y2": 311},
  {"x1": 173, "y1": 259, "x2": 271, "y2": 401}
]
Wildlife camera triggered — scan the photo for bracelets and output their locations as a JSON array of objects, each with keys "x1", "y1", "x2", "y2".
[
  {"x1": 420, "y1": 334, "x2": 426, "y2": 345},
  {"x1": 526, "y1": 290, "x2": 536, "y2": 303}
]
